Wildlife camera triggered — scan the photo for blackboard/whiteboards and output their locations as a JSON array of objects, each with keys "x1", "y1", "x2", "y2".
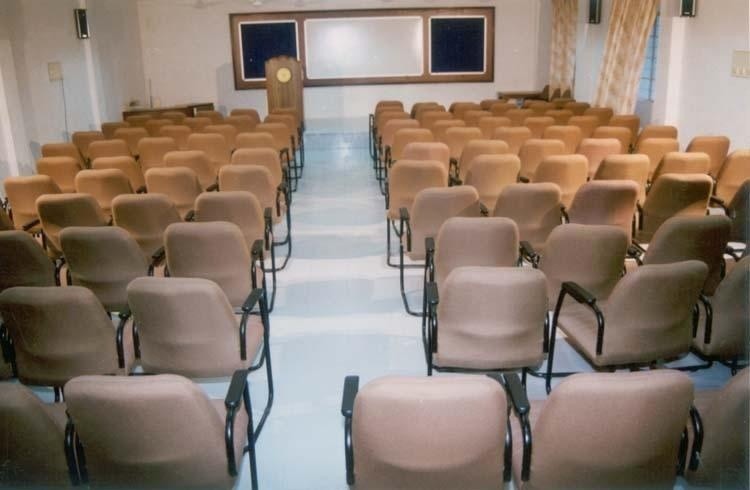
[{"x1": 229, "y1": 7, "x2": 494, "y2": 89}]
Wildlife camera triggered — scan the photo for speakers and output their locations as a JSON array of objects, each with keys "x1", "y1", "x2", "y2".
[
  {"x1": 588, "y1": 0, "x2": 601, "y2": 24},
  {"x1": 679, "y1": 0, "x2": 696, "y2": 17},
  {"x1": 75, "y1": 9, "x2": 88, "y2": 39}
]
